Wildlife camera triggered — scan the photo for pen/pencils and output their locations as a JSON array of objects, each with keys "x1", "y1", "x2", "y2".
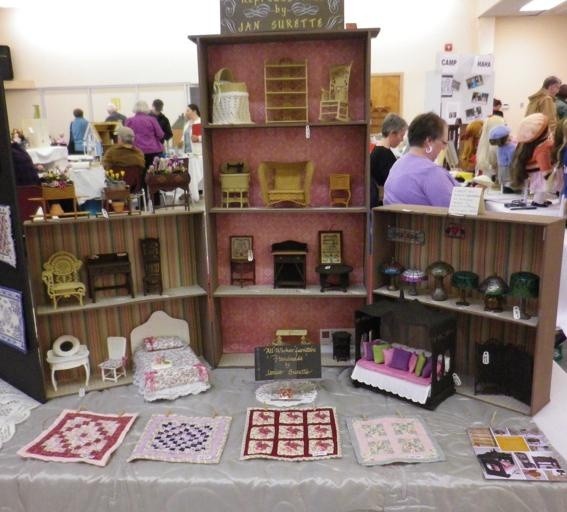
[{"x1": 510, "y1": 207, "x2": 536, "y2": 210}]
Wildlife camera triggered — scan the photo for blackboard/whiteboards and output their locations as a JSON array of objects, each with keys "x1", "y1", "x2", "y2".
[
  {"x1": 255, "y1": 343, "x2": 321, "y2": 381},
  {"x1": 220, "y1": 0, "x2": 344, "y2": 35}
]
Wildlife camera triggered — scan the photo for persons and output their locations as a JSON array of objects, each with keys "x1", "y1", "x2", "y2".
[
  {"x1": 68, "y1": 98, "x2": 202, "y2": 190},
  {"x1": 369, "y1": 75, "x2": 566, "y2": 215}
]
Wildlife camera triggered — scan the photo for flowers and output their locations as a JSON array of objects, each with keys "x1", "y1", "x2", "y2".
[
  {"x1": 105, "y1": 169, "x2": 126, "y2": 182},
  {"x1": 34, "y1": 164, "x2": 75, "y2": 188},
  {"x1": 145, "y1": 152, "x2": 187, "y2": 175}
]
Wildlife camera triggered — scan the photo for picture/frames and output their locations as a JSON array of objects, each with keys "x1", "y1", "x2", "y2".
[
  {"x1": 230, "y1": 235, "x2": 254, "y2": 260},
  {"x1": 318, "y1": 229, "x2": 344, "y2": 266}
]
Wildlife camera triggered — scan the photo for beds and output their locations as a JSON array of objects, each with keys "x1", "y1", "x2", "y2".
[{"x1": 130, "y1": 309, "x2": 212, "y2": 404}]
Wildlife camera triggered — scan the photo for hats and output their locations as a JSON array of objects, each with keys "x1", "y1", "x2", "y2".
[
  {"x1": 510, "y1": 112, "x2": 549, "y2": 145},
  {"x1": 488, "y1": 125, "x2": 511, "y2": 140}
]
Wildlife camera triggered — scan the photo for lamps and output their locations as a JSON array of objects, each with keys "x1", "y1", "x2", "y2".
[{"x1": 380, "y1": 257, "x2": 540, "y2": 320}]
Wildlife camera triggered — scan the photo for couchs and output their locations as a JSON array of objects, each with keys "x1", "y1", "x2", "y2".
[{"x1": 351, "y1": 300, "x2": 458, "y2": 411}]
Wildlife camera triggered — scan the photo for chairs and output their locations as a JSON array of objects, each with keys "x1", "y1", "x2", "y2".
[
  {"x1": 219, "y1": 159, "x2": 250, "y2": 209},
  {"x1": 97, "y1": 335, "x2": 128, "y2": 385},
  {"x1": 327, "y1": 174, "x2": 352, "y2": 208},
  {"x1": 468, "y1": 334, "x2": 516, "y2": 396},
  {"x1": 41, "y1": 249, "x2": 87, "y2": 312},
  {"x1": 317, "y1": 58, "x2": 356, "y2": 122},
  {"x1": 139, "y1": 235, "x2": 163, "y2": 297}
]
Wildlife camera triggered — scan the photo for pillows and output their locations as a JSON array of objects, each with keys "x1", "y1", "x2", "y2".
[
  {"x1": 144, "y1": 336, "x2": 185, "y2": 353},
  {"x1": 363, "y1": 340, "x2": 442, "y2": 378}
]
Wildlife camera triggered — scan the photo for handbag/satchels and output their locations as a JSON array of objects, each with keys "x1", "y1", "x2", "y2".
[{"x1": 67, "y1": 137, "x2": 75, "y2": 155}]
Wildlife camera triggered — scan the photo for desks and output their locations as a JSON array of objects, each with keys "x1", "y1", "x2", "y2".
[
  {"x1": 141, "y1": 172, "x2": 191, "y2": 213},
  {"x1": 276, "y1": 329, "x2": 309, "y2": 344},
  {"x1": 84, "y1": 250, "x2": 136, "y2": 304},
  {"x1": 0, "y1": 359, "x2": 565, "y2": 512},
  {"x1": 476, "y1": 183, "x2": 566, "y2": 347},
  {"x1": 220, "y1": 171, "x2": 251, "y2": 209},
  {"x1": 315, "y1": 265, "x2": 354, "y2": 293},
  {"x1": 230, "y1": 258, "x2": 256, "y2": 288},
  {"x1": 62, "y1": 153, "x2": 107, "y2": 208},
  {"x1": 40, "y1": 183, "x2": 78, "y2": 220},
  {"x1": 103, "y1": 185, "x2": 131, "y2": 214},
  {"x1": 208, "y1": 91, "x2": 257, "y2": 127},
  {"x1": 44, "y1": 333, "x2": 91, "y2": 393}
]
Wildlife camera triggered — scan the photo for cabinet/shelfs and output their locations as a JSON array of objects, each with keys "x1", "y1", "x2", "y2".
[
  {"x1": 186, "y1": 26, "x2": 382, "y2": 371},
  {"x1": 369, "y1": 202, "x2": 566, "y2": 420},
  {"x1": 264, "y1": 56, "x2": 310, "y2": 124},
  {"x1": 21, "y1": 202, "x2": 209, "y2": 405},
  {"x1": 92, "y1": 120, "x2": 124, "y2": 155},
  {"x1": 332, "y1": 330, "x2": 352, "y2": 363},
  {"x1": 256, "y1": 159, "x2": 315, "y2": 209},
  {"x1": 271, "y1": 239, "x2": 308, "y2": 289}
]
